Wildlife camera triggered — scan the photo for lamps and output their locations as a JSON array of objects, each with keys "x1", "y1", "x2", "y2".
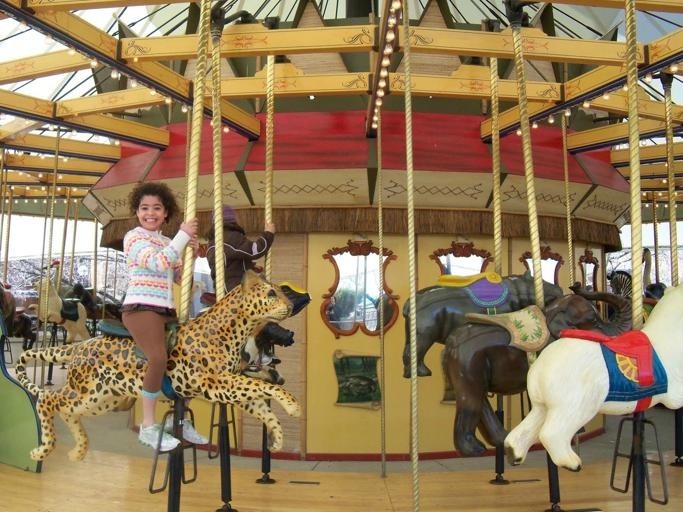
[
  {"x1": 371, "y1": 0, "x2": 405, "y2": 131},
  {"x1": 1, "y1": 110, "x2": 123, "y2": 147},
  {"x1": 7, "y1": 184, "x2": 78, "y2": 192},
  {"x1": 514, "y1": 61, "x2": 679, "y2": 137},
  {"x1": 5, "y1": 147, "x2": 68, "y2": 162},
  {"x1": 4, "y1": 198, "x2": 81, "y2": 204},
  {"x1": 66, "y1": 45, "x2": 229, "y2": 133},
  {"x1": 642, "y1": 186, "x2": 680, "y2": 208},
  {"x1": 17, "y1": 169, "x2": 64, "y2": 180}
]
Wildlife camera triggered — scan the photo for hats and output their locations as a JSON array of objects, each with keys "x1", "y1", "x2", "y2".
[{"x1": 211, "y1": 203, "x2": 236, "y2": 221}]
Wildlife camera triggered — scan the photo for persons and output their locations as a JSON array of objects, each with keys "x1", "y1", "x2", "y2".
[
  {"x1": 73, "y1": 284, "x2": 94, "y2": 309},
  {"x1": 206, "y1": 204, "x2": 276, "y2": 295},
  {"x1": 121, "y1": 183, "x2": 209, "y2": 451}
]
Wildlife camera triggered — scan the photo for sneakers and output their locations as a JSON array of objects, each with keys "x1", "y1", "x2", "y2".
[
  {"x1": 247, "y1": 352, "x2": 282, "y2": 372},
  {"x1": 138, "y1": 419, "x2": 209, "y2": 452}
]
